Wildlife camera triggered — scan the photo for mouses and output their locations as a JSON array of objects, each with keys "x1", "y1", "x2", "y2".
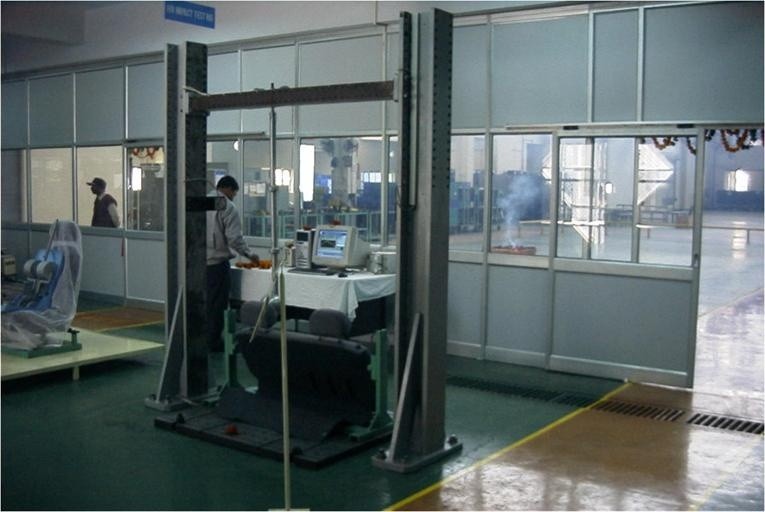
[{"x1": 338, "y1": 272, "x2": 348, "y2": 277}]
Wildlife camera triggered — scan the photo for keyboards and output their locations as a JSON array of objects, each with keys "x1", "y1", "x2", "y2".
[{"x1": 288, "y1": 269, "x2": 334, "y2": 276}]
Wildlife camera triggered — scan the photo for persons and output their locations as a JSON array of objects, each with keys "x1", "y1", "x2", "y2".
[
  {"x1": 206, "y1": 174, "x2": 260, "y2": 352},
  {"x1": 85, "y1": 177, "x2": 121, "y2": 228}
]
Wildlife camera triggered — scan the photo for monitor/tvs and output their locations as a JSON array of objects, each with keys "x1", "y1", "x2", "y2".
[{"x1": 312, "y1": 224, "x2": 370, "y2": 271}]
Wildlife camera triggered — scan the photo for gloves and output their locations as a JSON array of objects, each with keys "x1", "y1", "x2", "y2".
[{"x1": 247, "y1": 251, "x2": 260, "y2": 262}]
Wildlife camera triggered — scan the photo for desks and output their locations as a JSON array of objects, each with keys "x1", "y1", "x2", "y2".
[{"x1": 229, "y1": 262, "x2": 396, "y2": 342}]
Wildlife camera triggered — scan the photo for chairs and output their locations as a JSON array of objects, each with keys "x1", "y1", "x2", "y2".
[
  {"x1": 1, "y1": 221, "x2": 81, "y2": 334},
  {"x1": 234, "y1": 299, "x2": 377, "y2": 412}
]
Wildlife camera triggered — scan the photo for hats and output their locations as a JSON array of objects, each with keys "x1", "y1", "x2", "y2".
[{"x1": 86, "y1": 178, "x2": 106, "y2": 188}]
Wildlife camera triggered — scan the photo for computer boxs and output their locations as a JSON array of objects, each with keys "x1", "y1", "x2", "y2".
[{"x1": 295, "y1": 229, "x2": 315, "y2": 269}]
